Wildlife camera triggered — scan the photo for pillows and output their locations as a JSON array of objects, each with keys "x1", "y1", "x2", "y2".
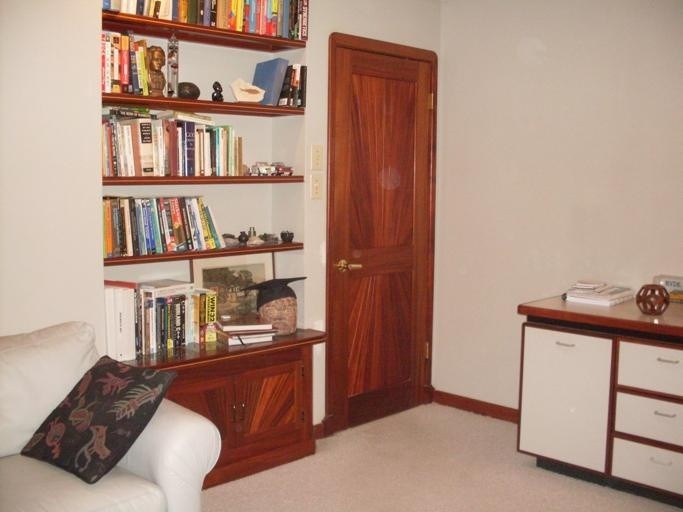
[{"x1": 18, "y1": 354, "x2": 179, "y2": 485}]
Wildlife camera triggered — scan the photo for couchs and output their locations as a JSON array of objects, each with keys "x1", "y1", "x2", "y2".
[{"x1": 1, "y1": 319, "x2": 227, "y2": 512}]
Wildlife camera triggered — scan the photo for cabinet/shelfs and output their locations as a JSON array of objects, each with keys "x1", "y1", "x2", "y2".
[
  {"x1": 115, "y1": 326, "x2": 328, "y2": 490},
  {"x1": 513, "y1": 280, "x2": 683, "y2": 506},
  {"x1": 103, "y1": 0, "x2": 312, "y2": 271}
]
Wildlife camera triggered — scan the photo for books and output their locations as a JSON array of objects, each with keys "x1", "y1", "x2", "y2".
[
  {"x1": 563, "y1": 280, "x2": 636, "y2": 308},
  {"x1": 102, "y1": 1, "x2": 310, "y2": 177},
  {"x1": 102, "y1": 276, "x2": 274, "y2": 363},
  {"x1": 102, "y1": 195, "x2": 225, "y2": 259}
]
